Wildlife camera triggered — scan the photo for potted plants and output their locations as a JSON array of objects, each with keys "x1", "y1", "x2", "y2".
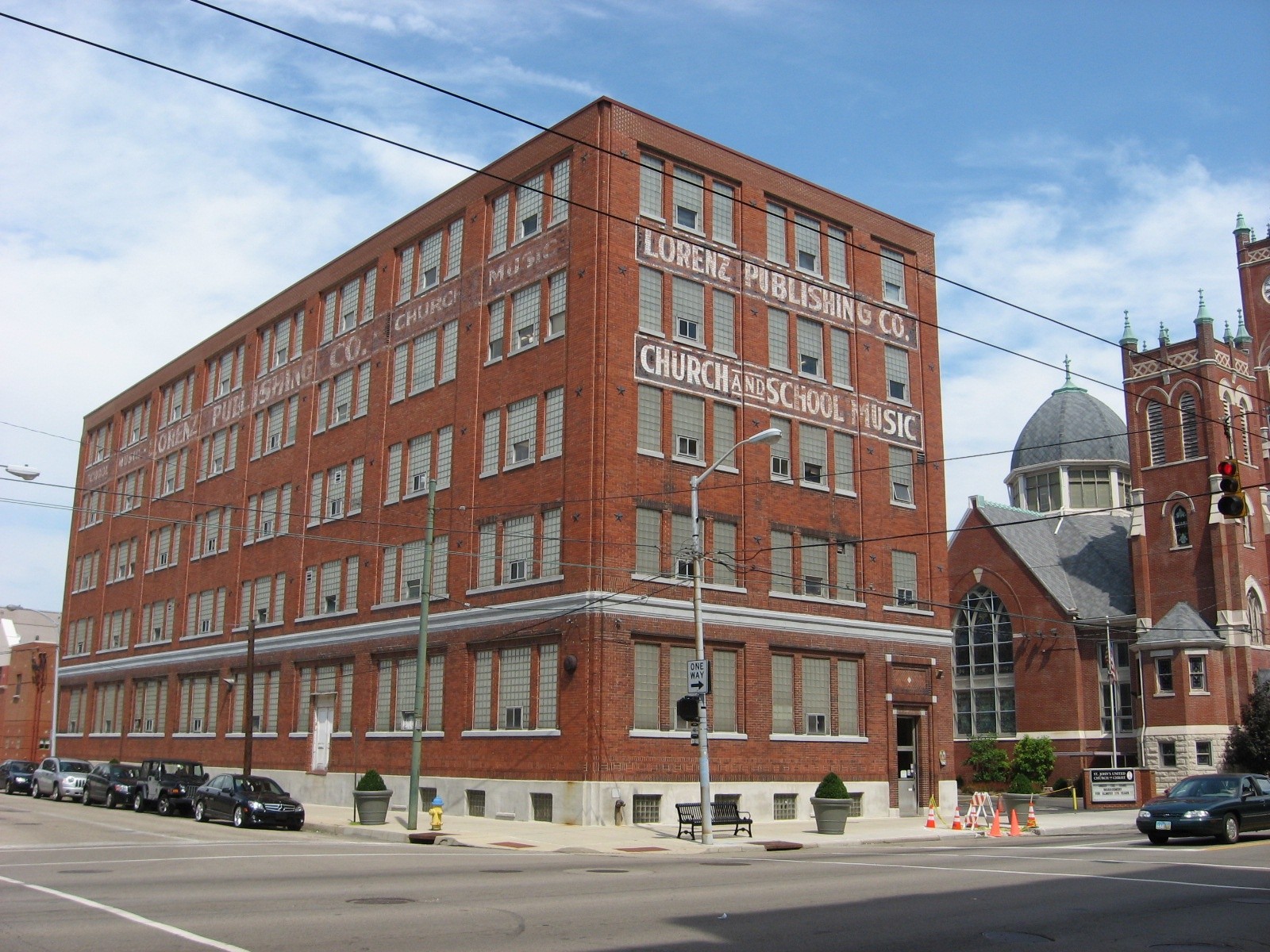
[
  {"x1": 810, "y1": 771, "x2": 854, "y2": 835},
  {"x1": 352, "y1": 769, "x2": 393, "y2": 825},
  {"x1": 1002, "y1": 772, "x2": 1041, "y2": 825}
]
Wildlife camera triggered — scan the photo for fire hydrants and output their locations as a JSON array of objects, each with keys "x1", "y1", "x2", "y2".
[{"x1": 428, "y1": 797, "x2": 443, "y2": 831}]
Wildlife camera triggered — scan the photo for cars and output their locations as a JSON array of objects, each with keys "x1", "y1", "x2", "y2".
[
  {"x1": 0, "y1": 758, "x2": 39, "y2": 795},
  {"x1": 193, "y1": 774, "x2": 307, "y2": 832},
  {"x1": 1137, "y1": 771, "x2": 1270, "y2": 844},
  {"x1": 80, "y1": 763, "x2": 143, "y2": 809}
]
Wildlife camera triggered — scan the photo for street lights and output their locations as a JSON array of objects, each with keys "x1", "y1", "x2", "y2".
[{"x1": 691, "y1": 428, "x2": 784, "y2": 846}]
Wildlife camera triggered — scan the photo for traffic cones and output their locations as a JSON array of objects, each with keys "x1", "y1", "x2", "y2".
[
  {"x1": 965, "y1": 803, "x2": 980, "y2": 827},
  {"x1": 1007, "y1": 809, "x2": 1022, "y2": 837},
  {"x1": 927, "y1": 805, "x2": 937, "y2": 828},
  {"x1": 952, "y1": 807, "x2": 963, "y2": 831},
  {"x1": 997, "y1": 794, "x2": 1006, "y2": 813},
  {"x1": 987, "y1": 810, "x2": 1002, "y2": 837},
  {"x1": 1025, "y1": 802, "x2": 1039, "y2": 829}
]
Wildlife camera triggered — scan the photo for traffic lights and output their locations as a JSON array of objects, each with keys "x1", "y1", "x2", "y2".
[{"x1": 1214, "y1": 459, "x2": 1246, "y2": 519}]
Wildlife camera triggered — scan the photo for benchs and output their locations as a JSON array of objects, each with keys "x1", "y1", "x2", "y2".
[{"x1": 675, "y1": 802, "x2": 753, "y2": 841}]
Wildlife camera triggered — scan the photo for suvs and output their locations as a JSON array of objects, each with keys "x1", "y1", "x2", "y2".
[
  {"x1": 133, "y1": 759, "x2": 209, "y2": 816},
  {"x1": 32, "y1": 756, "x2": 95, "y2": 801}
]
